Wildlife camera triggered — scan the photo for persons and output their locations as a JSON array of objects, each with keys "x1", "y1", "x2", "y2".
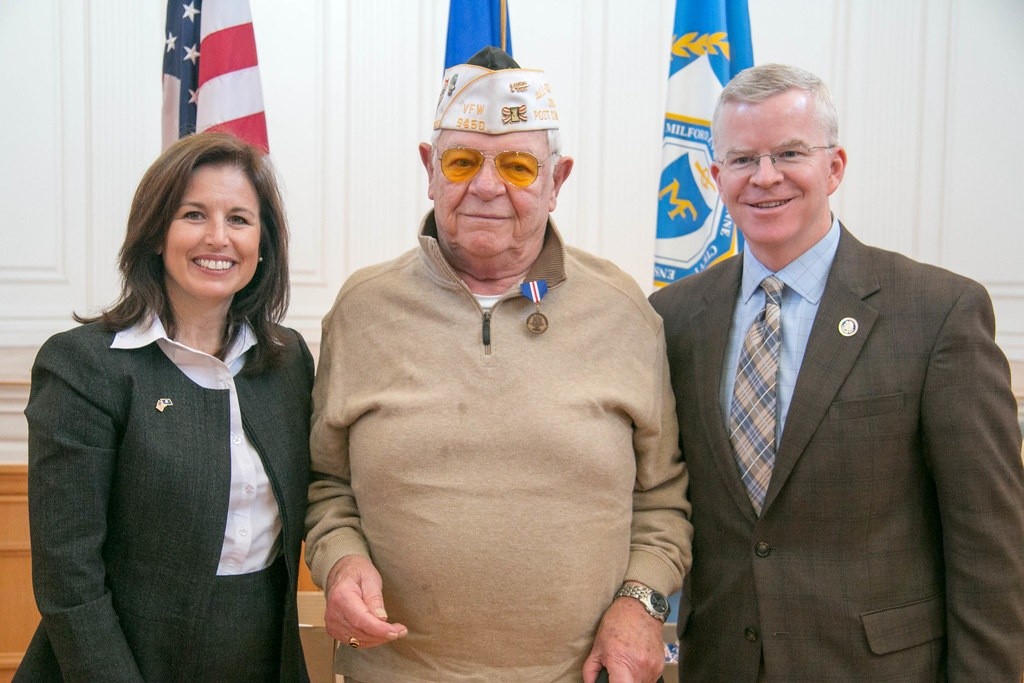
[
  {"x1": 641, "y1": 58, "x2": 1024, "y2": 683},
  {"x1": 299, "y1": 44, "x2": 696, "y2": 682},
  {"x1": 5, "y1": 128, "x2": 318, "y2": 682}
]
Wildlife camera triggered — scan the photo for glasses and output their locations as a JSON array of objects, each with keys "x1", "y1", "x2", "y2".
[
  {"x1": 439, "y1": 148, "x2": 554, "y2": 187},
  {"x1": 718, "y1": 145, "x2": 832, "y2": 175}
]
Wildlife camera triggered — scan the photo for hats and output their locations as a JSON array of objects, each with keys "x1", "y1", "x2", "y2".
[{"x1": 432, "y1": 46, "x2": 557, "y2": 135}]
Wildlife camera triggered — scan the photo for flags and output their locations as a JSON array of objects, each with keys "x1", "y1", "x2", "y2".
[
  {"x1": 156, "y1": 1, "x2": 272, "y2": 162},
  {"x1": 439, "y1": 0, "x2": 514, "y2": 80},
  {"x1": 649, "y1": 1, "x2": 757, "y2": 291}
]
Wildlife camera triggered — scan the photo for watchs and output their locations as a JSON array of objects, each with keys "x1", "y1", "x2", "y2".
[{"x1": 614, "y1": 582, "x2": 672, "y2": 624}]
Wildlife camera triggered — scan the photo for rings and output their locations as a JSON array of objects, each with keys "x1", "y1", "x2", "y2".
[{"x1": 348, "y1": 637, "x2": 360, "y2": 649}]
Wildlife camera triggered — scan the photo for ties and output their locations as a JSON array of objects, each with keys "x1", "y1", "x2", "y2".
[{"x1": 730, "y1": 275, "x2": 784, "y2": 517}]
